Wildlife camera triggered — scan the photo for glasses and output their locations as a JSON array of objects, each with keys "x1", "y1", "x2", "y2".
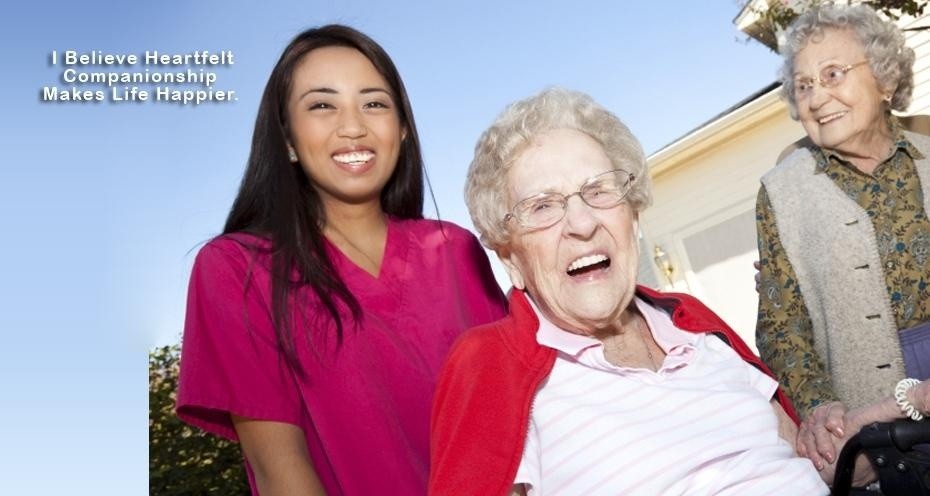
[
  {"x1": 499, "y1": 168, "x2": 635, "y2": 229},
  {"x1": 788, "y1": 60, "x2": 870, "y2": 100}
]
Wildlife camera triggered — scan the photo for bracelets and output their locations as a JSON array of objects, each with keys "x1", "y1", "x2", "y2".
[{"x1": 892, "y1": 378, "x2": 924, "y2": 421}]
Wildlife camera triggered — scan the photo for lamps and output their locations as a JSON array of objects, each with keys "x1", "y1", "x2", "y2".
[{"x1": 653, "y1": 245, "x2": 674, "y2": 287}]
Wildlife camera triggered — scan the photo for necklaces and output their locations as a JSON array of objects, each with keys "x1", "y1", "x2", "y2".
[
  {"x1": 332, "y1": 223, "x2": 376, "y2": 266},
  {"x1": 637, "y1": 321, "x2": 657, "y2": 371}
]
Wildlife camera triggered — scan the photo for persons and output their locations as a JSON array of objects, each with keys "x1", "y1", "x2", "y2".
[
  {"x1": 426, "y1": 84, "x2": 928, "y2": 496},
  {"x1": 173, "y1": 22, "x2": 512, "y2": 496},
  {"x1": 754, "y1": 4, "x2": 929, "y2": 489}
]
